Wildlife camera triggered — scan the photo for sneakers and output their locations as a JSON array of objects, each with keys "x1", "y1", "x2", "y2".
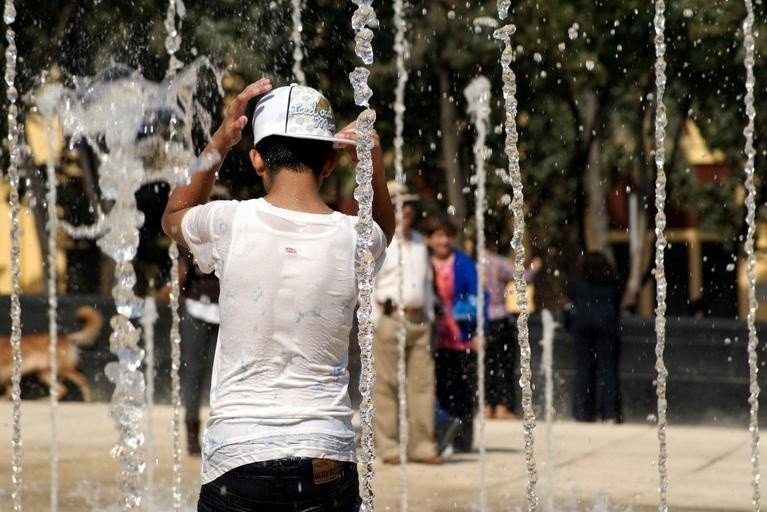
[
  {"x1": 383, "y1": 455, "x2": 443, "y2": 463},
  {"x1": 484, "y1": 403, "x2": 518, "y2": 419},
  {"x1": 434, "y1": 414, "x2": 463, "y2": 456}
]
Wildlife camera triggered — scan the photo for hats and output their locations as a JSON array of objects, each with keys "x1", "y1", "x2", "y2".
[
  {"x1": 386, "y1": 179, "x2": 420, "y2": 205},
  {"x1": 252, "y1": 83, "x2": 357, "y2": 147}
]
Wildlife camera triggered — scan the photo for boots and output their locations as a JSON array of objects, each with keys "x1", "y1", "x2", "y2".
[{"x1": 187, "y1": 420, "x2": 201, "y2": 454}]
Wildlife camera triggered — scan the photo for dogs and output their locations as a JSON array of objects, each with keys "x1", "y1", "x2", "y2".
[{"x1": 0, "y1": 304, "x2": 103, "y2": 403}]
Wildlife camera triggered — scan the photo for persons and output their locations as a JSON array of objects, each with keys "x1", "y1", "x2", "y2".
[
  {"x1": 175, "y1": 188, "x2": 231, "y2": 453},
  {"x1": 162, "y1": 78, "x2": 387, "y2": 512},
  {"x1": 561, "y1": 251, "x2": 624, "y2": 423},
  {"x1": 368, "y1": 180, "x2": 541, "y2": 470}
]
